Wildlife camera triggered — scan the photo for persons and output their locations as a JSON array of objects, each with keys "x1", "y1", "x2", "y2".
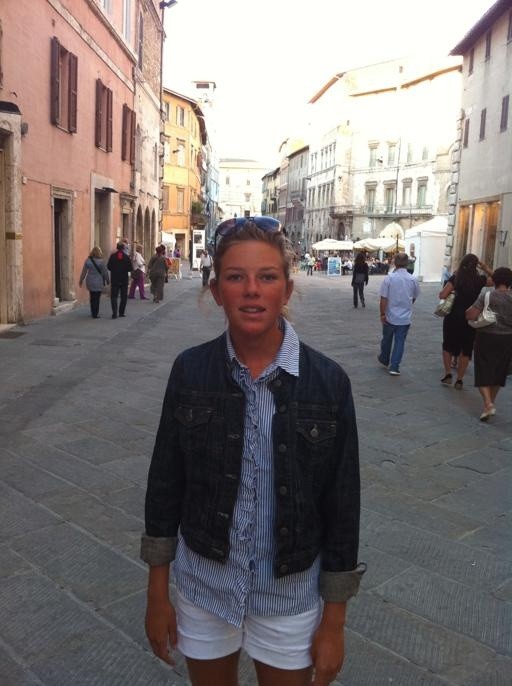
[
  {"x1": 304, "y1": 252, "x2": 369, "y2": 309},
  {"x1": 137, "y1": 216, "x2": 367, "y2": 686},
  {"x1": 378, "y1": 253, "x2": 419, "y2": 376},
  {"x1": 439, "y1": 254, "x2": 494, "y2": 390},
  {"x1": 149, "y1": 247, "x2": 168, "y2": 302},
  {"x1": 108, "y1": 243, "x2": 133, "y2": 319},
  {"x1": 79, "y1": 246, "x2": 110, "y2": 318},
  {"x1": 199, "y1": 250, "x2": 213, "y2": 287},
  {"x1": 407, "y1": 250, "x2": 415, "y2": 274},
  {"x1": 128, "y1": 245, "x2": 147, "y2": 300},
  {"x1": 465, "y1": 266, "x2": 512, "y2": 421},
  {"x1": 166, "y1": 248, "x2": 180, "y2": 257}
]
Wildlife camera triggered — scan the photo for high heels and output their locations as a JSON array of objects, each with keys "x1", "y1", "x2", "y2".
[
  {"x1": 455, "y1": 380, "x2": 464, "y2": 389},
  {"x1": 441, "y1": 374, "x2": 452, "y2": 384},
  {"x1": 480, "y1": 403, "x2": 496, "y2": 421}
]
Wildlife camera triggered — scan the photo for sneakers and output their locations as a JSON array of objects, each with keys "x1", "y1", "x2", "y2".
[
  {"x1": 449, "y1": 360, "x2": 457, "y2": 368},
  {"x1": 388, "y1": 370, "x2": 400, "y2": 375},
  {"x1": 119, "y1": 313, "x2": 126, "y2": 317},
  {"x1": 112, "y1": 314, "x2": 117, "y2": 318},
  {"x1": 376, "y1": 355, "x2": 388, "y2": 369}
]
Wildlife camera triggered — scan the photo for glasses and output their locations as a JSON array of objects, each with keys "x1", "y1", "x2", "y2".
[{"x1": 214, "y1": 216, "x2": 289, "y2": 255}]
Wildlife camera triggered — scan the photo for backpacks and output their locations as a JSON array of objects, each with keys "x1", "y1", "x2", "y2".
[{"x1": 355, "y1": 264, "x2": 366, "y2": 283}]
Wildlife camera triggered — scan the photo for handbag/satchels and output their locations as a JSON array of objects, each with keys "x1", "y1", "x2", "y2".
[
  {"x1": 131, "y1": 269, "x2": 141, "y2": 280},
  {"x1": 434, "y1": 270, "x2": 458, "y2": 317},
  {"x1": 468, "y1": 290, "x2": 499, "y2": 328}
]
